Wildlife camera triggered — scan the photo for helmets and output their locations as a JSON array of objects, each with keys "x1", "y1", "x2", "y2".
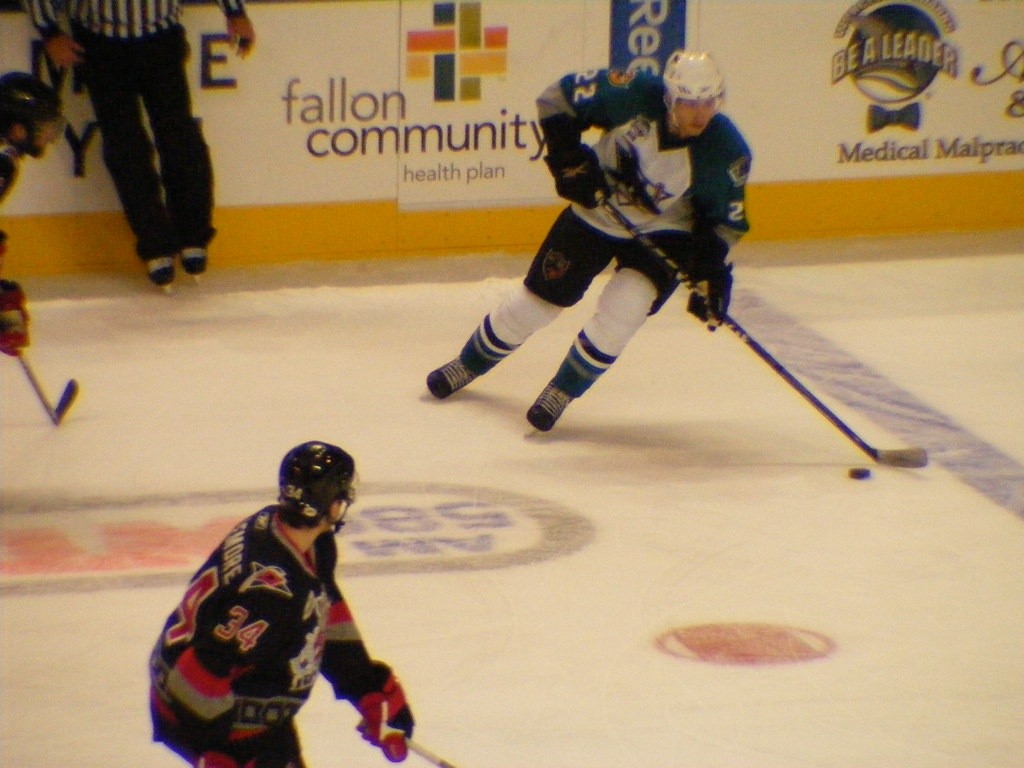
[
  {"x1": 0, "y1": 71, "x2": 60, "y2": 134},
  {"x1": 279, "y1": 441, "x2": 357, "y2": 531},
  {"x1": 662, "y1": 50, "x2": 724, "y2": 108}
]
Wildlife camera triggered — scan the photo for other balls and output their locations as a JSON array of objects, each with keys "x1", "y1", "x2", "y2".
[{"x1": 849, "y1": 468, "x2": 871, "y2": 480}]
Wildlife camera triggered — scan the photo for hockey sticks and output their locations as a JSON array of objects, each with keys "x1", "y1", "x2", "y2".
[
  {"x1": 18, "y1": 352, "x2": 80, "y2": 427},
  {"x1": 542, "y1": 152, "x2": 930, "y2": 469},
  {"x1": 355, "y1": 718, "x2": 455, "y2": 768}
]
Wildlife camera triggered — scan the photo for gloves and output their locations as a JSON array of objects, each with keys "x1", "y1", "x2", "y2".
[
  {"x1": 686, "y1": 262, "x2": 733, "y2": 332},
  {"x1": 349, "y1": 659, "x2": 415, "y2": 761},
  {"x1": 0, "y1": 280, "x2": 30, "y2": 361},
  {"x1": 544, "y1": 142, "x2": 612, "y2": 210}
]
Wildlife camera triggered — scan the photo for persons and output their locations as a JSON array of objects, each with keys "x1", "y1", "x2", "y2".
[
  {"x1": 0, "y1": 70, "x2": 66, "y2": 356},
  {"x1": 148, "y1": 441, "x2": 416, "y2": 768},
  {"x1": 22, "y1": 0, "x2": 256, "y2": 286},
  {"x1": 427, "y1": 50, "x2": 754, "y2": 432}
]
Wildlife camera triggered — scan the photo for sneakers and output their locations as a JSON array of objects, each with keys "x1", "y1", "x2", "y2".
[
  {"x1": 144, "y1": 243, "x2": 176, "y2": 294},
  {"x1": 420, "y1": 354, "x2": 479, "y2": 402},
  {"x1": 180, "y1": 242, "x2": 208, "y2": 282},
  {"x1": 526, "y1": 381, "x2": 575, "y2": 436}
]
